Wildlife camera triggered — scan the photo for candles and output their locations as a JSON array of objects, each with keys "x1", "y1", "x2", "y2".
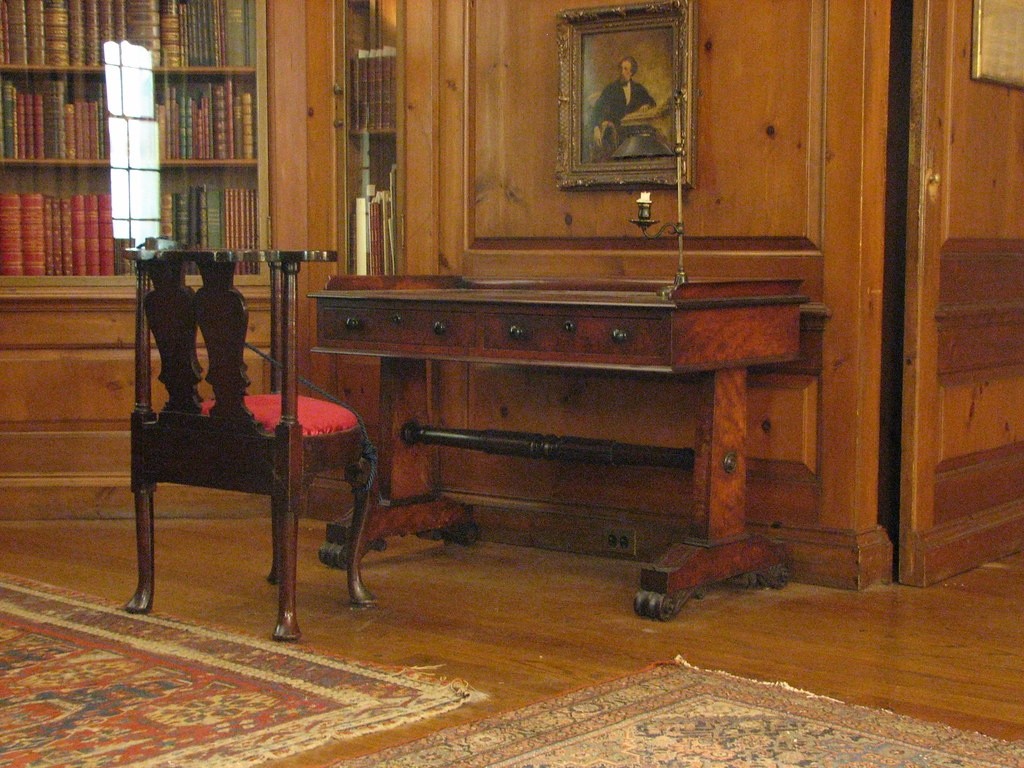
[{"x1": 639, "y1": 191, "x2": 650, "y2": 201}]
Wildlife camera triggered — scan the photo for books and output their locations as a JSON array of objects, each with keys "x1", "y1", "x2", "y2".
[
  {"x1": 0, "y1": 78, "x2": 254, "y2": 162},
  {"x1": 0, "y1": 0, "x2": 253, "y2": 66},
  {"x1": 0, "y1": 185, "x2": 257, "y2": 276},
  {"x1": 349, "y1": 47, "x2": 402, "y2": 279}
]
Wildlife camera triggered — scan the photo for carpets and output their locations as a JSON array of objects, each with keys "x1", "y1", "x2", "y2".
[
  {"x1": 0, "y1": 570, "x2": 492, "y2": 768},
  {"x1": 319, "y1": 652, "x2": 1024, "y2": 768}
]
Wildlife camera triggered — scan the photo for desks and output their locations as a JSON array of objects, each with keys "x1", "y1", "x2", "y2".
[{"x1": 305, "y1": 270, "x2": 811, "y2": 624}]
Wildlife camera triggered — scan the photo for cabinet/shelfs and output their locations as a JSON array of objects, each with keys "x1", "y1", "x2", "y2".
[{"x1": 0, "y1": 0, "x2": 273, "y2": 521}]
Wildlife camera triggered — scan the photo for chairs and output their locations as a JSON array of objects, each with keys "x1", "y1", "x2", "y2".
[{"x1": 129, "y1": 242, "x2": 385, "y2": 645}]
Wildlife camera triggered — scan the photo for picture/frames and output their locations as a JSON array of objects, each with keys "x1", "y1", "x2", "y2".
[{"x1": 554, "y1": 0, "x2": 697, "y2": 194}]
